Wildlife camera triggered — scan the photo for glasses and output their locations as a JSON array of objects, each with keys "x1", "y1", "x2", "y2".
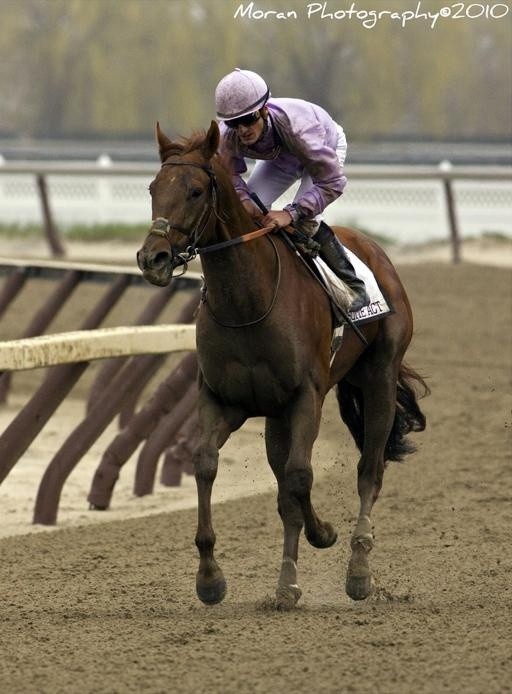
[{"x1": 224, "y1": 109, "x2": 262, "y2": 130}]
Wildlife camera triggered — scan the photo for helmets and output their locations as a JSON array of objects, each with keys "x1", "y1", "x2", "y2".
[{"x1": 215, "y1": 68, "x2": 271, "y2": 121}]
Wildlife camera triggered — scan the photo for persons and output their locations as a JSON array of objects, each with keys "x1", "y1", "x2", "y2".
[{"x1": 208, "y1": 66, "x2": 395, "y2": 330}]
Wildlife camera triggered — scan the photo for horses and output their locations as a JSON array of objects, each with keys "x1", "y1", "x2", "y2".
[{"x1": 136, "y1": 119, "x2": 430, "y2": 611}]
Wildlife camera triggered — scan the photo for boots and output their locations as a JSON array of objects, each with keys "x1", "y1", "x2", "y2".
[{"x1": 311, "y1": 221, "x2": 371, "y2": 312}]
